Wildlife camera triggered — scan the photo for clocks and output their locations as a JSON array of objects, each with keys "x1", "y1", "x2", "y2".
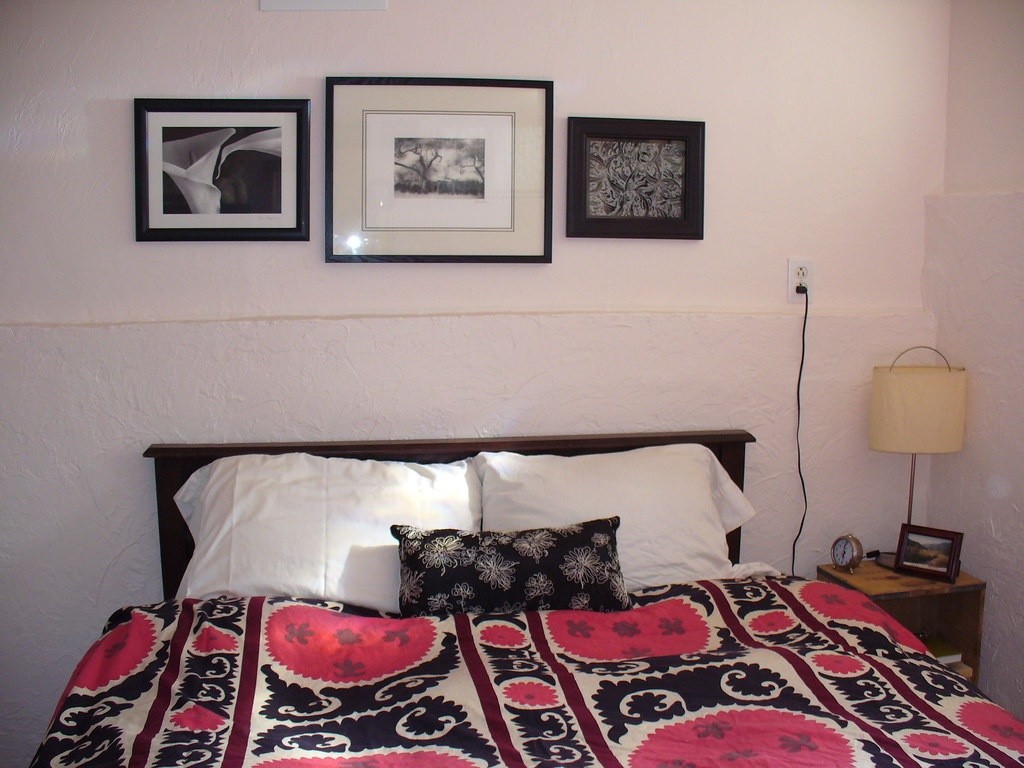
[{"x1": 830, "y1": 531, "x2": 863, "y2": 574}]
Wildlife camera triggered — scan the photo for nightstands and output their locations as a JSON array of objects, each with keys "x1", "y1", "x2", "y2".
[{"x1": 816, "y1": 562, "x2": 986, "y2": 688}]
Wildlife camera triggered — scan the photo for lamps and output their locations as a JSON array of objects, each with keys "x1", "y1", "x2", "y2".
[{"x1": 867, "y1": 346, "x2": 966, "y2": 568}]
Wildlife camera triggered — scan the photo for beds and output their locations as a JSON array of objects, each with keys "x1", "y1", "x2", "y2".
[{"x1": 27, "y1": 429, "x2": 1024, "y2": 768}]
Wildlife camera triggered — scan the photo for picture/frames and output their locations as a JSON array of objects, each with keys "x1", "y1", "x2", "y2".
[
  {"x1": 134, "y1": 97, "x2": 310, "y2": 242},
  {"x1": 326, "y1": 76, "x2": 554, "y2": 264},
  {"x1": 892, "y1": 523, "x2": 966, "y2": 585},
  {"x1": 565, "y1": 116, "x2": 704, "y2": 241}
]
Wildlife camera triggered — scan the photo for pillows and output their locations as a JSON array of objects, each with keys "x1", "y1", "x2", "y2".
[
  {"x1": 174, "y1": 452, "x2": 481, "y2": 615},
  {"x1": 389, "y1": 516, "x2": 633, "y2": 619},
  {"x1": 474, "y1": 443, "x2": 781, "y2": 593}
]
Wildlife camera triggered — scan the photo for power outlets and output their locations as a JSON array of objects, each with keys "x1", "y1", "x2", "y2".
[{"x1": 789, "y1": 259, "x2": 813, "y2": 303}]
